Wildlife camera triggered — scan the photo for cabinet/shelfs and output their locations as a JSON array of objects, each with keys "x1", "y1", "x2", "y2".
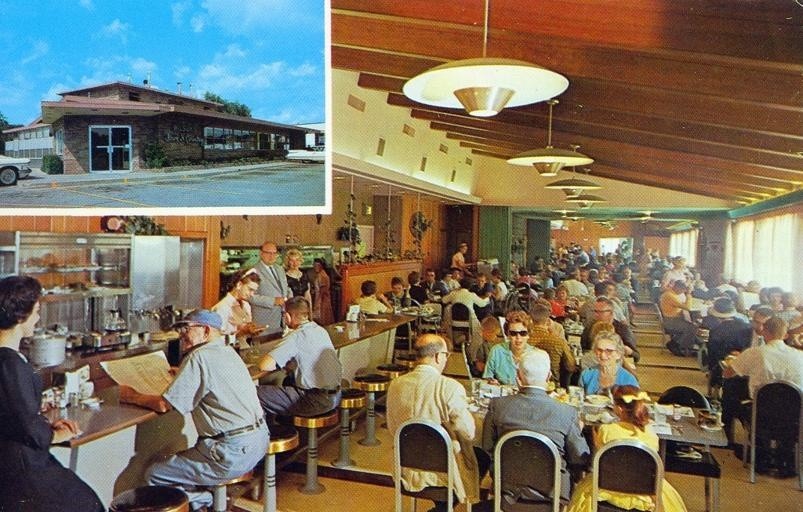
[
  {"x1": 0, "y1": 232, "x2": 134, "y2": 351},
  {"x1": 32, "y1": 347, "x2": 149, "y2": 391},
  {"x1": 40, "y1": 314, "x2": 418, "y2": 512},
  {"x1": 219, "y1": 244, "x2": 336, "y2": 312},
  {"x1": 332, "y1": 260, "x2": 422, "y2": 325}
]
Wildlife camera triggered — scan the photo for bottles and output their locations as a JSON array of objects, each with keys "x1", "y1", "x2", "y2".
[
  {"x1": 59, "y1": 393, "x2": 65, "y2": 408},
  {"x1": 673, "y1": 405, "x2": 680, "y2": 422},
  {"x1": 71, "y1": 393, "x2": 77, "y2": 408}
]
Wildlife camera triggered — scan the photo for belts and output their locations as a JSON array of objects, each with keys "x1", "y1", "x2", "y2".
[
  {"x1": 313, "y1": 385, "x2": 340, "y2": 395},
  {"x1": 202, "y1": 412, "x2": 265, "y2": 440}
]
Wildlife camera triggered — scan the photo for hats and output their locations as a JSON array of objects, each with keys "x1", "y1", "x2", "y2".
[
  {"x1": 169, "y1": 308, "x2": 222, "y2": 328},
  {"x1": 707, "y1": 297, "x2": 737, "y2": 318}
]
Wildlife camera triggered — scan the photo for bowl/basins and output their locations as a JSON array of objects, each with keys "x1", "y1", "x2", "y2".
[{"x1": 586, "y1": 395, "x2": 609, "y2": 407}]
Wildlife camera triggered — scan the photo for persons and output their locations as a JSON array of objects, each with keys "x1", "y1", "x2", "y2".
[
  {"x1": 578, "y1": 331, "x2": 639, "y2": 397},
  {"x1": 491, "y1": 268, "x2": 508, "y2": 313},
  {"x1": 581, "y1": 321, "x2": 637, "y2": 376},
  {"x1": 452, "y1": 243, "x2": 473, "y2": 276},
  {"x1": 580, "y1": 283, "x2": 630, "y2": 327},
  {"x1": 282, "y1": 249, "x2": 312, "y2": 309},
  {"x1": 516, "y1": 241, "x2": 638, "y2": 298},
  {"x1": 353, "y1": 280, "x2": 393, "y2": 315},
  {"x1": 707, "y1": 298, "x2": 752, "y2": 381},
  {"x1": 542, "y1": 287, "x2": 563, "y2": 317},
  {"x1": 469, "y1": 273, "x2": 493, "y2": 319},
  {"x1": 211, "y1": 268, "x2": 266, "y2": 351},
  {"x1": 527, "y1": 298, "x2": 564, "y2": 338},
  {"x1": 479, "y1": 310, "x2": 550, "y2": 398},
  {"x1": 579, "y1": 297, "x2": 640, "y2": 362},
  {"x1": 748, "y1": 280, "x2": 803, "y2": 350},
  {"x1": 662, "y1": 256, "x2": 694, "y2": 303},
  {"x1": 385, "y1": 276, "x2": 404, "y2": 307},
  {"x1": 482, "y1": 349, "x2": 590, "y2": 506},
  {"x1": 562, "y1": 384, "x2": 688, "y2": 512},
  {"x1": 440, "y1": 267, "x2": 469, "y2": 292},
  {"x1": 661, "y1": 280, "x2": 698, "y2": 356},
  {"x1": 247, "y1": 241, "x2": 293, "y2": 338},
  {"x1": 0, "y1": 275, "x2": 106, "y2": 512},
  {"x1": 313, "y1": 257, "x2": 335, "y2": 325},
  {"x1": 442, "y1": 278, "x2": 490, "y2": 327},
  {"x1": 527, "y1": 304, "x2": 577, "y2": 386},
  {"x1": 726, "y1": 307, "x2": 775, "y2": 356},
  {"x1": 419, "y1": 268, "x2": 450, "y2": 301},
  {"x1": 475, "y1": 316, "x2": 503, "y2": 372},
  {"x1": 385, "y1": 334, "x2": 490, "y2": 509},
  {"x1": 257, "y1": 296, "x2": 342, "y2": 417},
  {"x1": 118, "y1": 308, "x2": 271, "y2": 512},
  {"x1": 408, "y1": 271, "x2": 426, "y2": 306},
  {"x1": 728, "y1": 318, "x2": 803, "y2": 426}
]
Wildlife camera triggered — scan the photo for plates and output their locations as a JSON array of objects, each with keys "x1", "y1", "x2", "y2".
[
  {"x1": 654, "y1": 401, "x2": 695, "y2": 419},
  {"x1": 701, "y1": 424, "x2": 722, "y2": 433},
  {"x1": 549, "y1": 392, "x2": 581, "y2": 407},
  {"x1": 479, "y1": 399, "x2": 490, "y2": 410},
  {"x1": 645, "y1": 420, "x2": 672, "y2": 436},
  {"x1": 584, "y1": 401, "x2": 609, "y2": 409}
]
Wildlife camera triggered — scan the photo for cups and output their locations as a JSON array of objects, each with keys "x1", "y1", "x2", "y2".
[{"x1": 655, "y1": 409, "x2": 667, "y2": 425}]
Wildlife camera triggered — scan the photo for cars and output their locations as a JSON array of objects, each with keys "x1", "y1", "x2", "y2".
[{"x1": 0, "y1": 155, "x2": 32, "y2": 187}]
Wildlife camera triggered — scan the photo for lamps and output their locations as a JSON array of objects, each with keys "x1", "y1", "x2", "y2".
[
  {"x1": 507, "y1": 99, "x2": 594, "y2": 177},
  {"x1": 566, "y1": 168, "x2": 610, "y2": 211},
  {"x1": 402, "y1": 0, "x2": 569, "y2": 116},
  {"x1": 544, "y1": 144, "x2": 604, "y2": 197}
]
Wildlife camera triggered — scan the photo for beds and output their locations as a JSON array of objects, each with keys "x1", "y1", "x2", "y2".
[{"x1": 456, "y1": 376, "x2": 728, "y2": 512}]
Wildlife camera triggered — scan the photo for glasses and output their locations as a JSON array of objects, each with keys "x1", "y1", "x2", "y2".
[
  {"x1": 262, "y1": 250, "x2": 277, "y2": 254},
  {"x1": 439, "y1": 351, "x2": 450, "y2": 358},
  {"x1": 598, "y1": 348, "x2": 617, "y2": 353},
  {"x1": 509, "y1": 330, "x2": 527, "y2": 336},
  {"x1": 594, "y1": 309, "x2": 610, "y2": 313},
  {"x1": 175, "y1": 324, "x2": 203, "y2": 333}
]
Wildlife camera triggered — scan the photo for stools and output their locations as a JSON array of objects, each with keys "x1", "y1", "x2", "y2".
[
  {"x1": 330, "y1": 388, "x2": 366, "y2": 468},
  {"x1": 198, "y1": 470, "x2": 252, "y2": 512},
  {"x1": 258, "y1": 426, "x2": 300, "y2": 512},
  {"x1": 293, "y1": 409, "x2": 338, "y2": 495},
  {"x1": 109, "y1": 486, "x2": 189, "y2": 512},
  {"x1": 395, "y1": 354, "x2": 417, "y2": 368},
  {"x1": 377, "y1": 363, "x2": 409, "y2": 429},
  {"x1": 352, "y1": 374, "x2": 392, "y2": 446}
]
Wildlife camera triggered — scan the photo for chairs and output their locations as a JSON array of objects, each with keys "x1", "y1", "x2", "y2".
[
  {"x1": 569, "y1": 438, "x2": 665, "y2": 512},
  {"x1": 519, "y1": 281, "x2": 532, "y2": 312},
  {"x1": 742, "y1": 379, "x2": 803, "y2": 492},
  {"x1": 653, "y1": 302, "x2": 693, "y2": 358},
  {"x1": 421, "y1": 303, "x2": 442, "y2": 335},
  {"x1": 450, "y1": 302, "x2": 472, "y2": 353},
  {"x1": 656, "y1": 385, "x2": 722, "y2": 512},
  {"x1": 394, "y1": 320, "x2": 417, "y2": 358},
  {"x1": 401, "y1": 296, "x2": 421, "y2": 308},
  {"x1": 462, "y1": 340, "x2": 484, "y2": 379},
  {"x1": 394, "y1": 418, "x2": 490, "y2": 512},
  {"x1": 495, "y1": 429, "x2": 568, "y2": 512}
]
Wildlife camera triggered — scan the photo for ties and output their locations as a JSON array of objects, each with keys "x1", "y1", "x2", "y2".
[{"x1": 758, "y1": 337, "x2": 763, "y2": 346}]
